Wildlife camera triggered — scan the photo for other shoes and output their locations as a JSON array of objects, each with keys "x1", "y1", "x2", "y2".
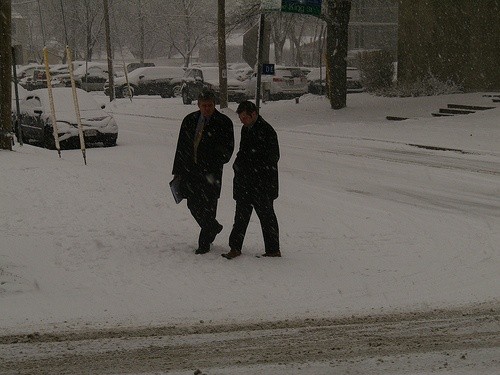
[
  {"x1": 195, "y1": 245, "x2": 210, "y2": 254},
  {"x1": 256, "y1": 250, "x2": 281, "y2": 258},
  {"x1": 203, "y1": 224, "x2": 223, "y2": 245},
  {"x1": 221, "y1": 250, "x2": 241, "y2": 259}
]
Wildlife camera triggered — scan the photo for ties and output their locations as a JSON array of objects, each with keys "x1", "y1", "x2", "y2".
[{"x1": 194, "y1": 119, "x2": 205, "y2": 160}]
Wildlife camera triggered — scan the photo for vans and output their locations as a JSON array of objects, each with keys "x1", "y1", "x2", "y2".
[{"x1": 182, "y1": 62, "x2": 202, "y2": 70}]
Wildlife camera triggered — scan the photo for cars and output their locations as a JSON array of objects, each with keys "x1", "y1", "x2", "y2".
[
  {"x1": 243, "y1": 65, "x2": 309, "y2": 101},
  {"x1": 233, "y1": 69, "x2": 246, "y2": 80},
  {"x1": 80, "y1": 72, "x2": 117, "y2": 92},
  {"x1": 300, "y1": 67, "x2": 312, "y2": 77},
  {"x1": 126, "y1": 62, "x2": 155, "y2": 73},
  {"x1": 63, "y1": 65, "x2": 103, "y2": 87},
  {"x1": 215, "y1": 82, "x2": 249, "y2": 103},
  {"x1": 103, "y1": 66, "x2": 190, "y2": 98},
  {"x1": 237, "y1": 69, "x2": 256, "y2": 82},
  {"x1": 216, "y1": 62, "x2": 232, "y2": 71},
  {"x1": 11, "y1": 65, "x2": 56, "y2": 83},
  {"x1": 20, "y1": 69, "x2": 65, "y2": 91},
  {"x1": 180, "y1": 66, "x2": 247, "y2": 105},
  {"x1": 307, "y1": 66, "x2": 366, "y2": 95},
  {"x1": 49, "y1": 65, "x2": 70, "y2": 75},
  {"x1": 13, "y1": 88, "x2": 118, "y2": 149},
  {"x1": 10, "y1": 96, "x2": 25, "y2": 133}
]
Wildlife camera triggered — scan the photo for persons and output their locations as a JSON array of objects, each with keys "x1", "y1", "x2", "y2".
[
  {"x1": 221, "y1": 101, "x2": 281, "y2": 260},
  {"x1": 171, "y1": 91, "x2": 235, "y2": 254}
]
